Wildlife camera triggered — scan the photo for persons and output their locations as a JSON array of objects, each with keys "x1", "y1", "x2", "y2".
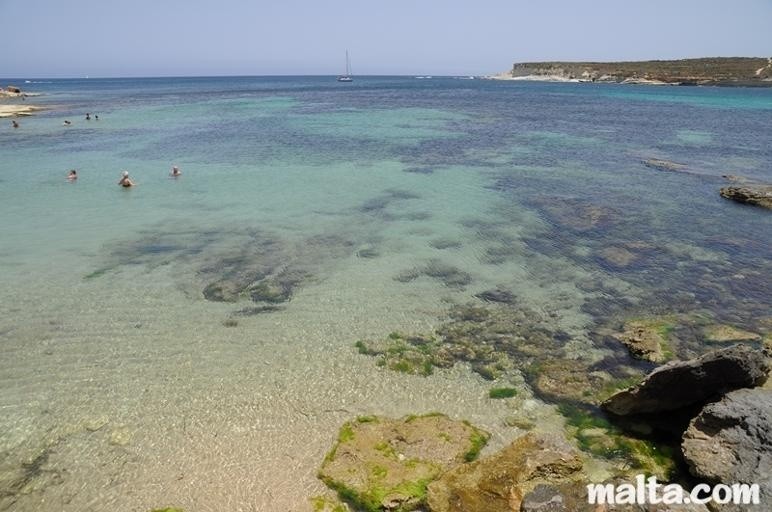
[
  {"x1": 95, "y1": 115, "x2": 99, "y2": 120},
  {"x1": 64, "y1": 170, "x2": 77, "y2": 181},
  {"x1": 117, "y1": 171, "x2": 136, "y2": 186},
  {"x1": 12, "y1": 121, "x2": 20, "y2": 128},
  {"x1": 170, "y1": 166, "x2": 182, "y2": 176},
  {"x1": 86, "y1": 113, "x2": 91, "y2": 120},
  {"x1": 63, "y1": 121, "x2": 68, "y2": 126},
  {"x1": 67, "y1": 121, "x2": 72, "y2": 126}
]
